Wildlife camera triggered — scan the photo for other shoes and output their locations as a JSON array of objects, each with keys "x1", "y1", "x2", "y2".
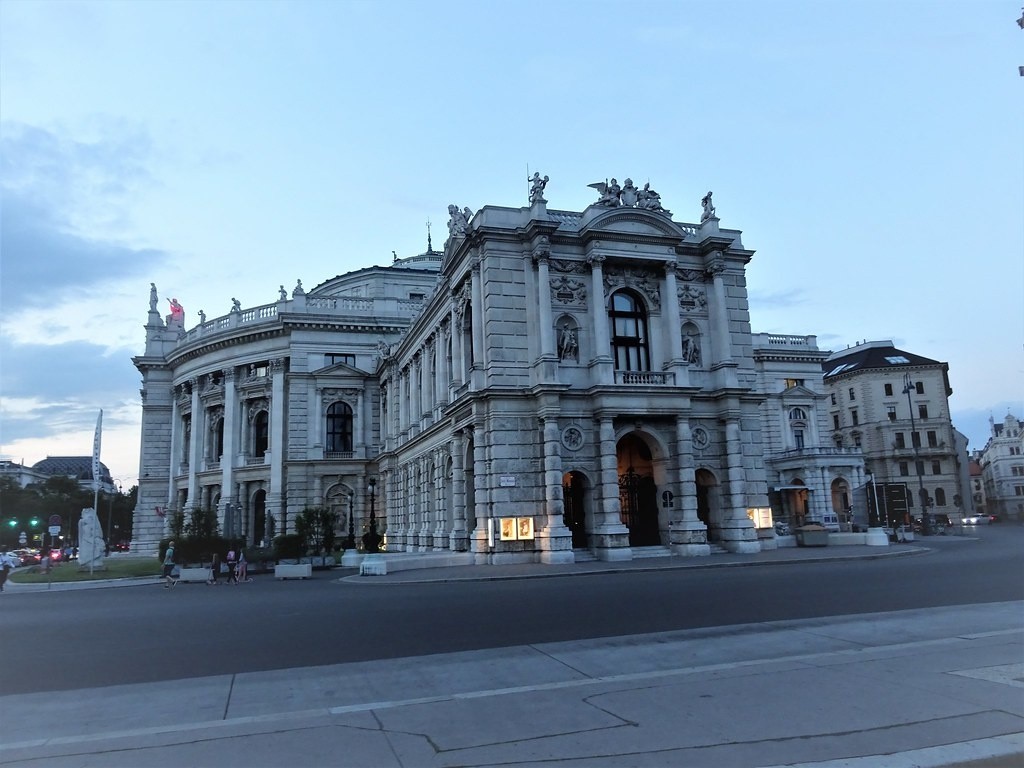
[
  {"x1": 235, "y1": 582, "x2": 238, "y2": 584},
  {"x1": 224, "y1": 582, "x2": 229, "y2": 584},
  {"x1": 173, "y1": 580, "x2": 178, "y2": 586},
  {"x1": 164, "y1": 586, "x2": 169, "y2": 590},
  {"x1": 1, "y1": 588, "x2": 5, "y2": 592}
]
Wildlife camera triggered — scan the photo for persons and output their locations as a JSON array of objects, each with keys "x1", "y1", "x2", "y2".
[
  {"x1": 279, "y1": 285, "x2": 288, "y2": 301},
  {"x1": 59, "y1": 545, "x2": 78, "y2": 564},
  {"x1": 682, "y1": 330, "x2": 700, "y2": 364},
  {"x1": 230, "y1": 297, "x2": 241, "y2": 312},
  {"x1": 166, "y1": 297, "x2": 185, "y2": 314},
  {"x1": 236, "y1": 548, "x2": 248, "y2": 582},
  {"x1": 528, "y1": 172, "x2": 549, "y2": 203},
  {"x1": 558, "y1": 323, "x2": 578, "y2": 360},
  {"x1": 181, "y1": 383, "x2": 188, "y2": 394},
  {"x1": 292, "y1": 279, "x2": 306, "y2": 298},
  {"x1": 147, "y1": 282, "x2": 158, "y2": 313},
  {"x1": 203, "y1": 373, "x2": 214, "y2": 392},
  {"x1": 198, "y1": 310, "x2": 206, "y2": 323},
  {"x1": 160, "y1": 540, "x2": 179, "y2": 590},
  {"x1": 206, "y1": 553, "x2": 221, "y2": 585},
  {"x1": 224, "y1": 548, "x2": 239, "y2": 585},
  {"x1": 700, "y1": 191, "x2": 720, "y2": 222},
  {"x1": 248, "y1": 364, "x2": 257, "y2": 376},
  {"x1": 633, "y1": 183, "x2": 665, "y2": 211},
  {"x1": 0, "y1": 544, "x2": 16, "y2": 592},
  {"x1": 587, "y1": 177, "x2": 623, "y2": 207}
]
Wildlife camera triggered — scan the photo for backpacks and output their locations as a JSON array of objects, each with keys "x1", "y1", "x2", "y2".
[{"x1": 168, "y1": 548, "x2": 178, "y2": 563}]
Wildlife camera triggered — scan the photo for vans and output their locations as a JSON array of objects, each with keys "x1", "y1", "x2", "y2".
[{"x1": 794, "y1": 512, "x2": 842, "y2": 534}]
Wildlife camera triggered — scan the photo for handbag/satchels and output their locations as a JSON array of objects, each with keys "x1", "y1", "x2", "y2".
[{"x1": 4, "y1": 565, "x2": 10, "y2": 574}]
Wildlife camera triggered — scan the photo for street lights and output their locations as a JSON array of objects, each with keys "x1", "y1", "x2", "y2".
[
  {"x1": 902, "y1": 373, "x2": 933, "y2": 536},
  {"x1": 362, "y1": 477, "x2": 382, "y2": 552},
  {"x1": 107, "y1": 478, "x2": 122, "y2": 540},
  {"x1": 347, "y1": 490, "x2": 355, "y2": 548}
]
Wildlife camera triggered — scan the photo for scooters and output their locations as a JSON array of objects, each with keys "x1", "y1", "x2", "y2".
[
  {"x1": 205, "y1": 563, "x2": 222, "y2": 585},
  {"x1": 235, "y1": 561, "x2": 254, "y2": 584}
]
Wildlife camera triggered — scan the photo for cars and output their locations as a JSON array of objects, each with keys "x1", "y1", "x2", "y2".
[
  {"x1": 0, "y1": 546, "x2": 79, "y2": 567},
  {"x1": 117, "y1": 540, "x2": 130, "y2": 550},
  {"x1": 961, "y1": 513, "x2": 992, "y2": 525}
]
